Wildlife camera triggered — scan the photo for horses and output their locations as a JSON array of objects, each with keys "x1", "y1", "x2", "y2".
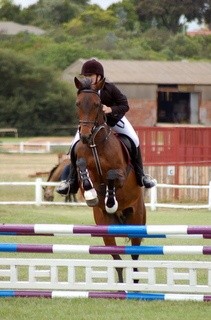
[
  {"x1": 67, "y1": 76, "x2": 146, "y2": 294},
  {"x1": 42, "y1": 158, "x2": 80, "y2": 203}
]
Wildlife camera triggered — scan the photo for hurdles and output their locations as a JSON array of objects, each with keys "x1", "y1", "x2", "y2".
[{"x1": 0, "y1": 224, "x2": 211, "y2": 301}]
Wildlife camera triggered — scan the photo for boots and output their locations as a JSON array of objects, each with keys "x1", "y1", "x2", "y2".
[
  {"x1": 135, "y1": 146, "x2": 156, "y2": 188},
  {"x1": 56, "y1": 151, "x2": 77, "y2": 194}
]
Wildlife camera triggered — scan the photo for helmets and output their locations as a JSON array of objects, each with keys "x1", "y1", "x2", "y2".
[{"x1": 80, "y1": 59, "x2": 104, "y2": 77}]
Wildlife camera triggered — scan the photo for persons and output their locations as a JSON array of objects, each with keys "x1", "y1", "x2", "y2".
[{"x1": 58, "y1": 59, "x2": 156, "y2": 193}]
